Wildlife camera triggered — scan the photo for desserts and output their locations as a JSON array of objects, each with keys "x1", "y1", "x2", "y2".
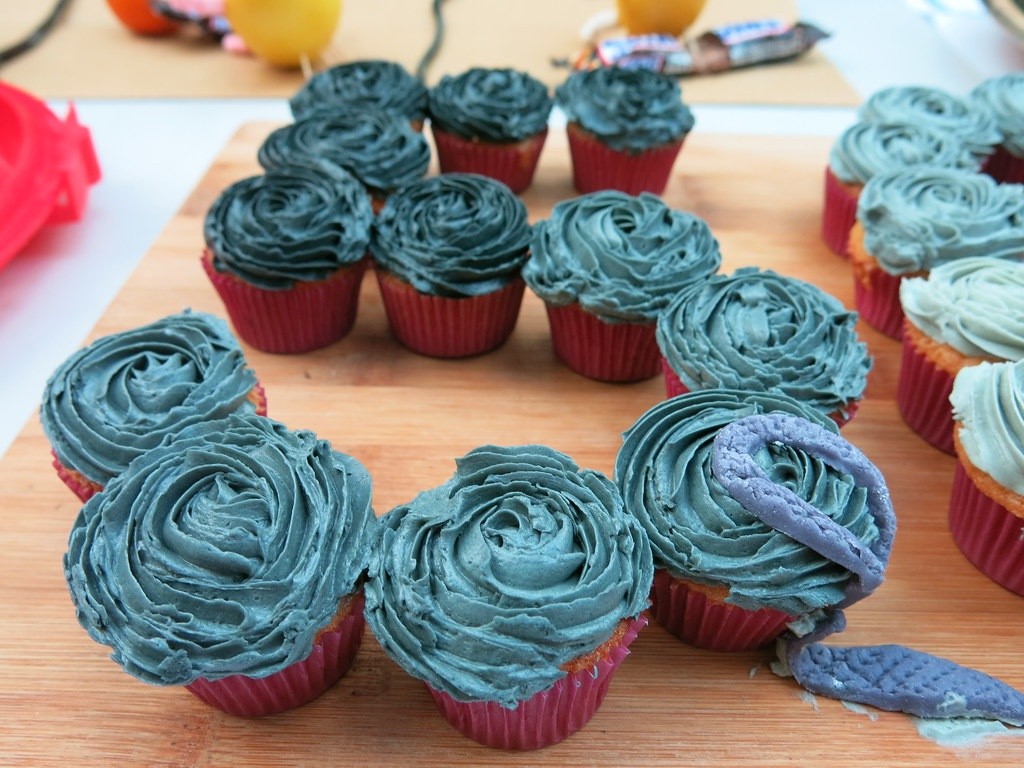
[{"x1": 39, "y1": 57, "x2": 1024, "y2": 752}]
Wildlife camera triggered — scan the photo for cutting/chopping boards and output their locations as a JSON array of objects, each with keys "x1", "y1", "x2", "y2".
[{"x1": 1, "y1": 119, "x2": 1024, "y2": 768}]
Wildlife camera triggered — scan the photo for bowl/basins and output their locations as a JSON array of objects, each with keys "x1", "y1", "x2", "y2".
[
  {"x1": 613, "y1": 0, "x2": 708, "y2": 39},
  {"x1": 224, "y1": 0, "x2": 342, "y2": 70},
  {"x1": 106, "y1": 1, "x2": 191, "y2": 37}
]
